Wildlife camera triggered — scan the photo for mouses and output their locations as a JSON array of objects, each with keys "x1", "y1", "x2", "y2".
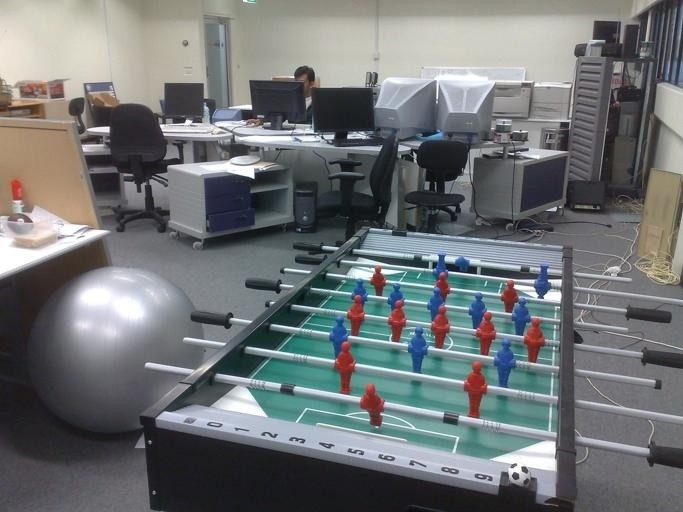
[{"x1": 211, "y1": 128, "x2": 228, "y2": 135}]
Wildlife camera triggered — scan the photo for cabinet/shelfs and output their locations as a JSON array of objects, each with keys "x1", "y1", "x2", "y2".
[
  {"x1": 168, "y1": 159, "x2": 295, "y2": 250},
  {"x1": 81, "y1": 145, "x2": 126, "y2": 215}
]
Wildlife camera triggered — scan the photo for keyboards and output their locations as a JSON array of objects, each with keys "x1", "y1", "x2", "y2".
[{"x1": 161, "y1": 127, "x2": 214, "y2": 133}]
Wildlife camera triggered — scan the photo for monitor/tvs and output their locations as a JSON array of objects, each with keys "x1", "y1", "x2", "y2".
[
  {"x1": 310, "y1": 86, "x2": 375, "y2": 137},
  {"x1": 436, "y1": 75, "x2": 496, "y2": 144},
  {"x1": 375, "y1": 76, "x2": 436, "y2": 140},
  {"x1": 162, "y1": 82, "x2": 204, "y2": 126},
  {"x1": 249, "y1": 79, "x2": 307, "y2": 131}
]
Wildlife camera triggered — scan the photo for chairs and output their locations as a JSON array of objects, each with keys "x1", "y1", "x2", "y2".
[
  {"x1": 410, "y1": 148, "x2": 461, "y2": 221},
  {"x1": 110, "y1": 103, "x2": 180, "y2": 233},
  {"x1": 318, "y1": 129, "x2": 399, "y2": 247},
  {"x1": 69, "y1": 97, "x2": 86, "y2": 134},
  {"x1": 405, "y1": 140, "x2": 468, "y2": 233}
]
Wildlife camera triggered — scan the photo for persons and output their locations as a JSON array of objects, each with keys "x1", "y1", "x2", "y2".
[{"x1": 246, "y1": 66, "x2": 316, "y2": 127}]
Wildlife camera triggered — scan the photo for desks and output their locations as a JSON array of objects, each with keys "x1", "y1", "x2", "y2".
[
  {"x1": 87, "y1": 123, "x2": 234, "y2": 162},
  {"x1": 401, "y1": 132, "x2": 525, "y2": 159},
  {"x1": 0, "y1": 216, "x2": 111, "y2": 280},
  {"x1": 213, "y1": 121, "x2": 412, "y2": 227}
]
